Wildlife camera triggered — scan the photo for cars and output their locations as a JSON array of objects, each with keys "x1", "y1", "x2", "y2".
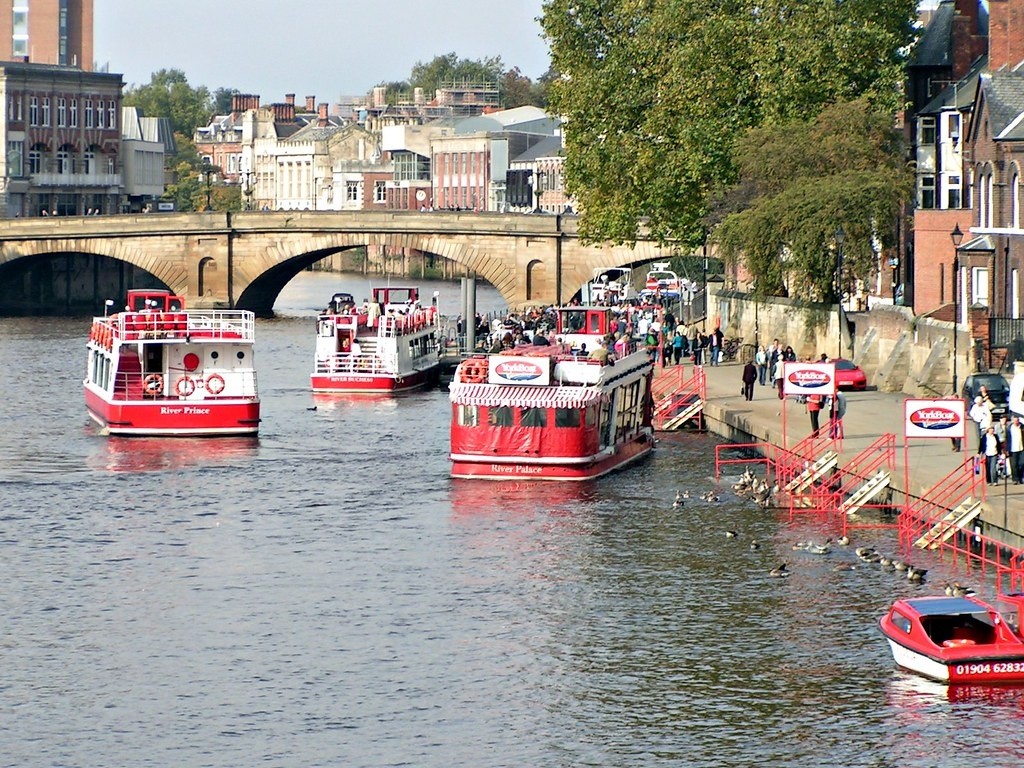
[{"x1": 962, "y1": 373, "x2": 1011, "y2": 417}]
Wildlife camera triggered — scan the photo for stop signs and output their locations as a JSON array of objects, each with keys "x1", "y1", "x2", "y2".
[{"x1": 645, "y1": 279, "x2": 657, "y2": 291}]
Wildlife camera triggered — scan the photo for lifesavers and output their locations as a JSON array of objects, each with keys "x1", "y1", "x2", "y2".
[
  {"x1": 205, "y1": 374, "x2": 225, "y2": 395},
  {"x1": 175, "y1": 376, "x2": 196, "y2": 396},
  {"x1": 324, "y1": 355, "x2": 383, "y2": 372},
  {"x1": 90, "y1": 322, "x2": 112, "y2": 350},
  {"x1": 144, "y1": 374, "x2": 164, "y2": 396},
  {"x1": 462, "y1": 360, "x2": 485, "y2": 384},
  {"x1": 943, "y1": 639, "x2": 976, "y2": 647}
]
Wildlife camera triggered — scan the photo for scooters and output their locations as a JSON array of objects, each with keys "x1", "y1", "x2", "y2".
[{"x1": 996, "y1": 440, "x2": 1011, "y2": 479}]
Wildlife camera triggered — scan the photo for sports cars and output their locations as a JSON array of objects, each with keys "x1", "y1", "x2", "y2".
[{"x1": 820, "y1": 358, "x2": 867, "y2": 391}]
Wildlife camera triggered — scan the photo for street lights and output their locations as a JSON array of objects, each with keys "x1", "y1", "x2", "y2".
[
  {"x1": 835, "y1": 225, "x2": 845, "y2": 359},
  {"x1": 527, "y1": 171, "x2": 547, "y2": 216},
  {"x1": 951, "y1": 222, "x2": 964, "y2": 395},
  {"x1": 238, "y1": 172, "x2": 258, "y2": 211}
]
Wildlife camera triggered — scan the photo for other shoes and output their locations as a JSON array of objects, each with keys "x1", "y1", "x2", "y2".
[
  {"x1": 987, "y1": 482, "x2": 992, "y2": 486},
  {"x1": 994, "y1": 483, "x2": 998, "y2": 485}
]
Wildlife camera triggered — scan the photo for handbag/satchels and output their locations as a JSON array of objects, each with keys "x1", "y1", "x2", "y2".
[
  {"x1": 741, "y1": 386, "x2": 744, "y2": 395},
  {"x1": 997, "y1": 456, "x2": 1012, "y2": 480},
  {"x1": 975, "y1": 456, "x2": 980, "y2": 474},
  {"x1": 690, "y1": 353, "x2": 696, "y2": 361},
  {"x1": 718, "y1": 351, "x2": 724, "y2": 363}
]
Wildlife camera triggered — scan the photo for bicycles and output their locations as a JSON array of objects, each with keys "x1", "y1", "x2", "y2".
[{"x1": 719, "y1": 337, "x2": 743, "y2": 360}]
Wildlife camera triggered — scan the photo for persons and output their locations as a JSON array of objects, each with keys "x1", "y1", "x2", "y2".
[
  {"x1": 742, "y1": 338, "x2": 847, "y2": 439},
  {"x1": 87, "y1": 207, "x2": 99, "y2": 215},
  {"x1": 41, "y1": 210, "x2": 58, "y2": 216},
  {"x1": 142, "y1": 206, "x2": 151, "y2": 213},
  {"x1": 323, "y1": 281, "x2": 726, "y2": 366},
  {"x1": 943, "y1": 384, "x2": 1024, "y2": 487},
  {"x1": 263, "y1": 203, "x2": 269, "y2": 211},
  {"x1": 15, "y1": 210, "x2": 20, "y2": 217}
]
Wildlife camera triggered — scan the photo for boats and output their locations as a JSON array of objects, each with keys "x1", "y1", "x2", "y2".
[
  {"x1": 84, "y1": 288, "x2": 263, "y2": 437},
  {"x1": 310, "y1": 286, "x2": 442, "y2": 395},
  {"x1": 580, "y1": 267, "x2": 641, "y2": 306},
  {"x1": 447, "y1": 306, "x2": 656, "y2": 482},
  {"x1": 639, "y1": 262, "x2": 697, "y2": 305}
]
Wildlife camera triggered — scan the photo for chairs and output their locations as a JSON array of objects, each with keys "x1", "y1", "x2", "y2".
[
  {"x1": 502, "y1": 342, "x2": 604, "y2": 368},
  {"x1": 329, "y1": 312, "x2": 433, "y2": 336},
  {"x1": 113, "y1": 315, "x2": 241, "y2": 342}
]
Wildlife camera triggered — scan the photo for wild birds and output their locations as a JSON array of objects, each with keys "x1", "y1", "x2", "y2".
[
  {"x1": 673, "y1": 466, "x2": 785, "y2": 506},
  {"x1": 725, "y1": 531, "x2": 978, "y2": 598}
]
